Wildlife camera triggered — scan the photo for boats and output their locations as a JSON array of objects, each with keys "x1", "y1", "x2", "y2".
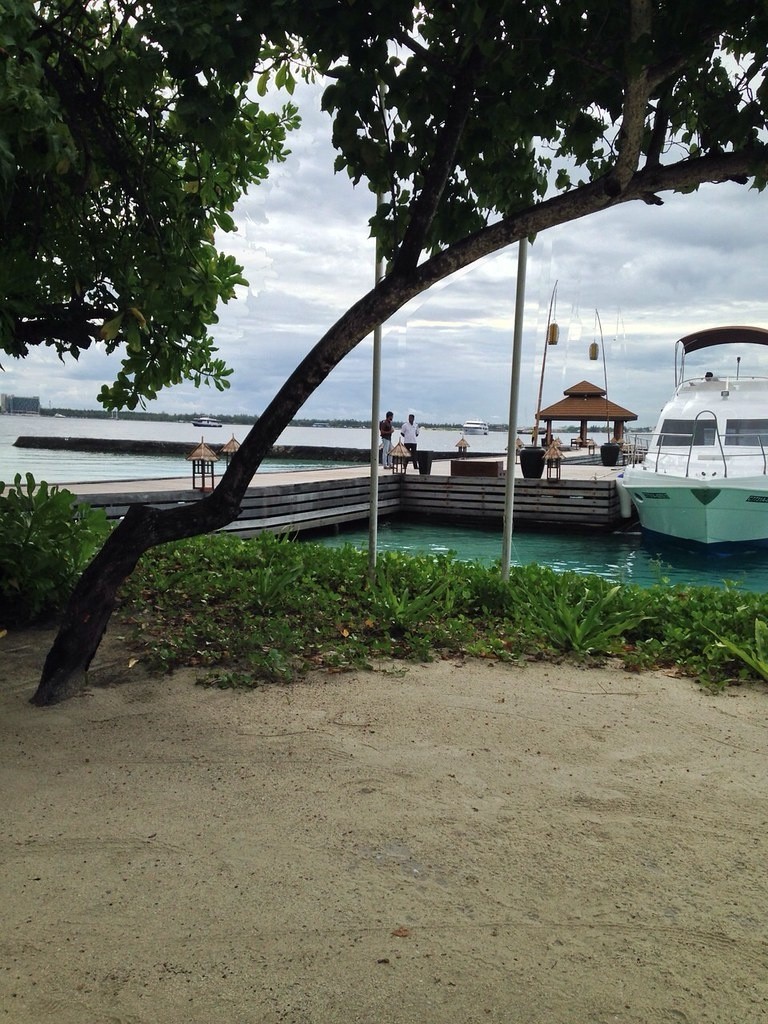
[
  {"x1": 620, "y1": 324, "x2": 768, "y2": 555},
  {"x1": 516, "y1": 426, "x2": 547, "y2": 434},
  {"x1": 55, "y1": 413, "x2": 67, "y2": 418},
  {"x1": 191, "y1": 415, "x2": 223, "y2": 428},
  {"x1": 462, "y1": 421, "x2": 489, "y2": 436}
]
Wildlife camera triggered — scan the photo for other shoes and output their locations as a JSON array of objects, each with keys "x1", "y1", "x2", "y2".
[
  {"x1": 384, "y1": 466, "x2": 391, "y2": 469},
  {"x1": 389, "y1": 466, "x2": 396, "y2": 469},
  {"x1": 414, "y1": 467, "x2": 419, "y2": 469},
  {"x1": 403, "y1": 467, "x2": 407, "y2": 469}
]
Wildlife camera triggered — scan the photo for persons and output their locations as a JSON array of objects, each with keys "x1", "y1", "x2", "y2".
[
  {"x1": 401, "y1": 414, "x2": 419, "y2": 469},
  {"x1": 531, "y1": 427, "x2": 535, "y2": 445},
  {"x1": 381, "y1": 411, "x2": 395, "y2": 469},
  {"x1": 689, "y1": 371, "x2": 718, "y2": 386}
]
